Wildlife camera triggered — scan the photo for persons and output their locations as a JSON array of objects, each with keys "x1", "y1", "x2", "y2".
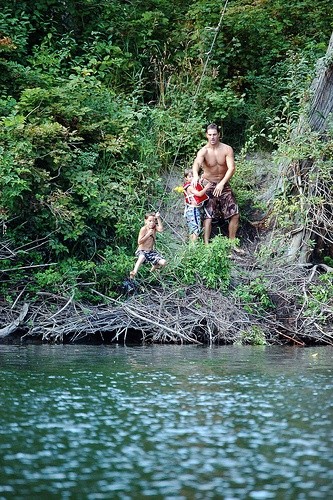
[
  {"x1": 183, "y1": 169, "x2": 212, "y2": 243},
  {"x1": 190, "y1": 123, "x2": 244, "y2": 255},
  {"x1": 130, "y1": 211, "x2": 166, "y2": 279}
]
